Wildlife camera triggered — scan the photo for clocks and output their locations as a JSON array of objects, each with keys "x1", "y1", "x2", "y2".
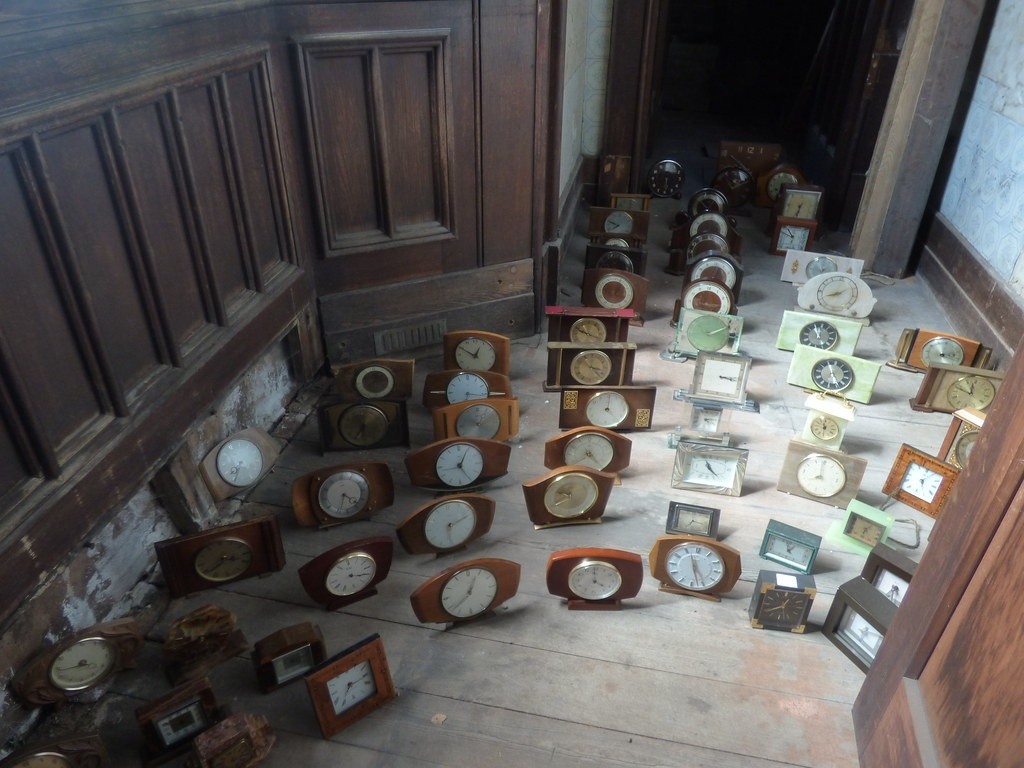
[
  {"x1": 747, "y1": 569, "x2": 818, "y2": 634},
  {"x1": 315, "y1": 139, "x2": 1005, "y2": 559},
  {"x1": 410, "y1": 557, "x2": 521, "y2": 634},
  {"x1": 133, "y1": 673, "x2": 241, "y2": 768},
  {"x1": 303, "y1": 631, "x2": 397, "y2": 742},
  {"x1": 186, "y1": 711, "x2": 278, "y2": 768},
  {"x1": 157, "y1": 604, "x2": 251, "y2": 689},
  {"x1": 154, "y1": 513, "x2": 286, "y2": 599},
  {"x1": 758, "y1": 519, "x2": 822, "y2": 576},
  {"x1": 647, "y1": 535, "x2": 742, "y2": 603},
  {"x1": 10, "y1": 616, "x2": 146, "y2": 708},
  {"x1": 0, "y1": 729, "x2": 112, "y2": 768},
  {"x1": 545, "y1": 548, "x2": 643, "y2": 611},
  {"x1": 821, "y1": 575, "x2": 898, "y2": 675},
  {"x1": 861, "y1": 542, "x2": 919, "y2": 607},
  {"x1": 198, "y1": 426, "x2": 283, "y2": 504},
  {"x1": 297, "y1": 535, "x2": 393, "y2": 613},
  {"x1": 291, "y1": 461, "x2": 395, "y2": 532},
  {"x1": 250, "y1": 621, "x2": 328, "y2": 694}
]
